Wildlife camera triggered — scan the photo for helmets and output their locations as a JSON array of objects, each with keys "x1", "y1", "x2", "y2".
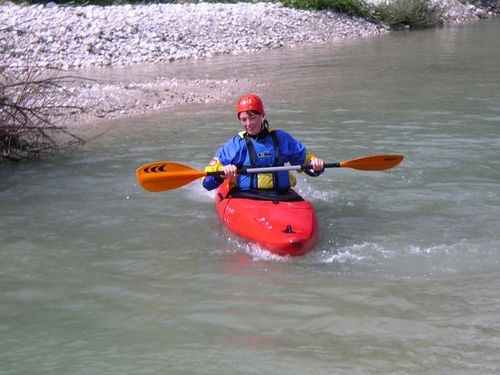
[{"x1": 236, "y1": 94, "x2": 265, "y2": 119}]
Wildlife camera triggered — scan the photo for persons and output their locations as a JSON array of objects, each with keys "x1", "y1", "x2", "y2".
[{"x1": 201, "y1": 93, "x2": 324, "y2": 192}]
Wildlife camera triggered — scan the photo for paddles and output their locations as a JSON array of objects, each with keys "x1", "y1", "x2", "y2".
[{"x1": 136, "y1": 153, "x2": 404, "y2": 192}]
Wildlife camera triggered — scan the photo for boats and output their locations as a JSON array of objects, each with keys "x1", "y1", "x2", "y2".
[{"x1": 215, "y1": 175, "x2": 319, "y2": 256}]
